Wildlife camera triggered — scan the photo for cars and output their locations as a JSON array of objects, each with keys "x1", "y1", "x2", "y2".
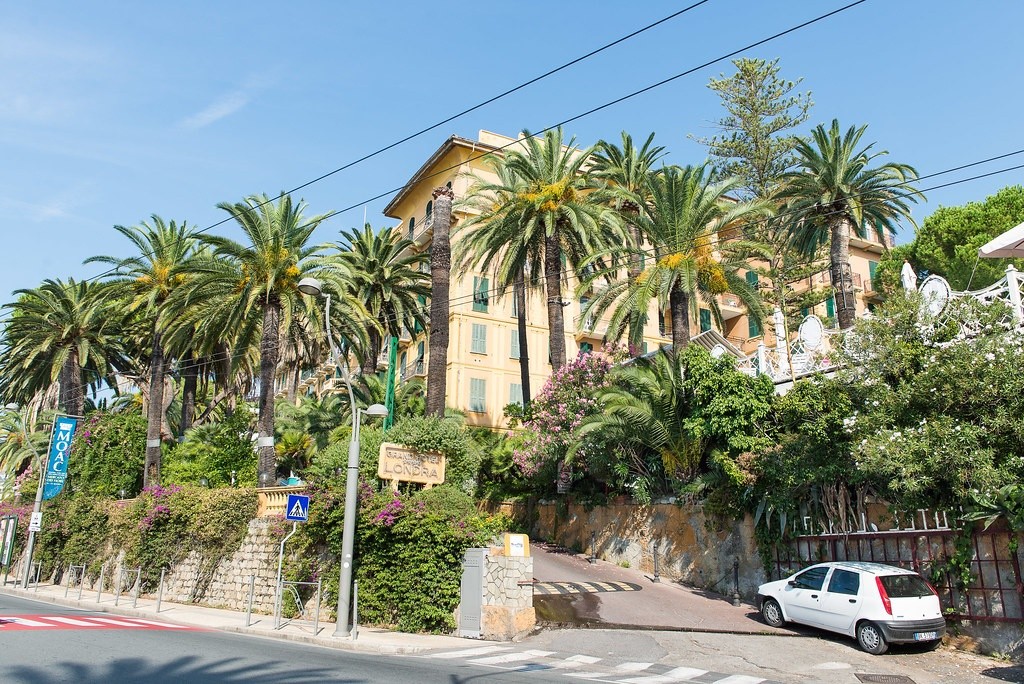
[{"x1": 756, "y1": 561, "x2": 946, "y2": 655}]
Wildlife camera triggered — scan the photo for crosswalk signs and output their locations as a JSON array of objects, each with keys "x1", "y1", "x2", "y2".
[{"x1": 286, "y1": 494, "x2": 310, "y2": 521}]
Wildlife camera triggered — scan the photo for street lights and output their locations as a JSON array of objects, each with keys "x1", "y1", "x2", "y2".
[
  {"x1": 298, "y1": 275, "x2": 389, "y2": 636},
  {"x1": 5, "y1": 402, "x2": 46, "y2": 592}
]
